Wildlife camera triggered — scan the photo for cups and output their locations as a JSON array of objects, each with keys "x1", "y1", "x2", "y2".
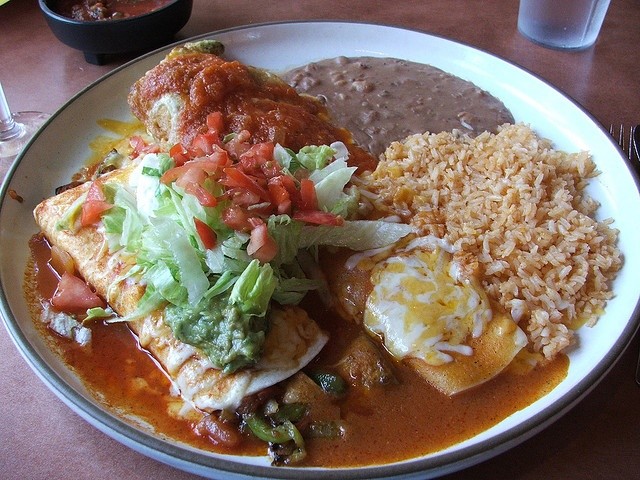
[{"x1": 515, "y1": 1, "x2": 613, "y2": 53}]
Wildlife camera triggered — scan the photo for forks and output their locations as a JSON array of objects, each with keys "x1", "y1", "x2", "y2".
[{"x1": 558, "y1": 121, "x2": 635, "y2": 434}]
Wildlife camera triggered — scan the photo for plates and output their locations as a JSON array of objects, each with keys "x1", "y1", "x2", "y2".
[{"x1": 0, "y1": 19, "x2": 640, "y2": 479}]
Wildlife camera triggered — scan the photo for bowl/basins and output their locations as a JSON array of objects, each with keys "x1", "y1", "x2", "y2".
[{"x1": 38, "y1": 0, "x2": 193, "y2": 66}]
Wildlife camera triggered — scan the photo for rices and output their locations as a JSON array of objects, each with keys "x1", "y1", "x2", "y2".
[{"x1": 364, "y1": 122, "x2": 622, "y2": 361}]
